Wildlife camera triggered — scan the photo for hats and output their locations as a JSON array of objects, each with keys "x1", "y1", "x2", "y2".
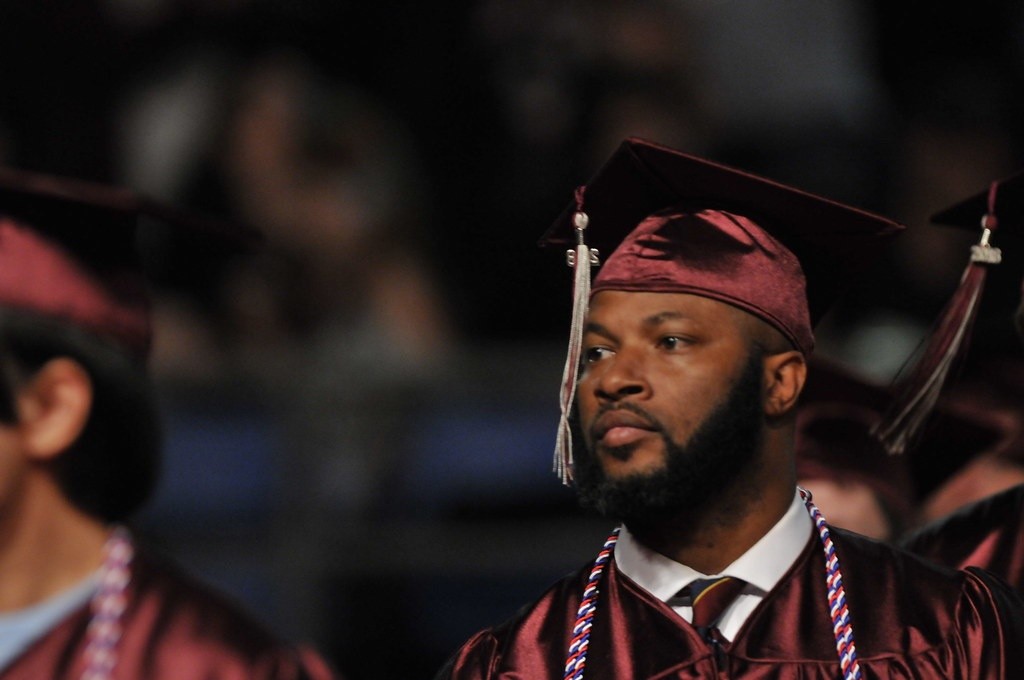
[
  {"x1": 2, "y1": 167, "x2": 268, "y2": 354},
  {"x1": 532, "y1": 138, "x2": 916, "y2": 490},
  {"x1": 863, "y1": 168, "x2": 1024, "y2": 457}
]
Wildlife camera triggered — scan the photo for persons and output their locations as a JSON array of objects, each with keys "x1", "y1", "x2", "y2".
[
  {"x1": 0, "y1": 162, "x2": 342, "y2": 680},
  {"x1": 424, "y1": 137, "x2": 1024, "y2": 680}
]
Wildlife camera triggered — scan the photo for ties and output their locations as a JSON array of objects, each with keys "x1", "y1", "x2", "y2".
[{"x1": 684, "y1": 576, "x2": 749, "y2": 671}]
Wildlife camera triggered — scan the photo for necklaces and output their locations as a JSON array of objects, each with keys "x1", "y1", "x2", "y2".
[
  {"x1": 565, "y1": 489, "x2": 862, "y2": 680},
  {"x1": 79, "y1": 523, "x2": 137, "y2": 680}
]
